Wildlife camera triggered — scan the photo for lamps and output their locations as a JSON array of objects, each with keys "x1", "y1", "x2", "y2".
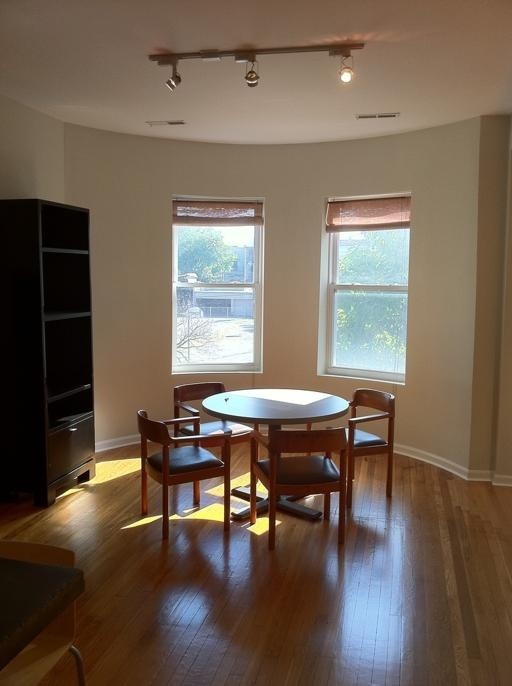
[{"x1": 149, "y1": 42, "x2": 366, "y2": 91}]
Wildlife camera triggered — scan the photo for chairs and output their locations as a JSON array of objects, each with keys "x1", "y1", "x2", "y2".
[
  {"x1": 0, "y1": 542, "x2": 85, "y2": 685},
  {"x1": 326, "y1": 389, "x2": 395, "y2": 508},
  {"x1": 174, "y1": 382, "x2": 260, "y2": 484},
  {"x1": 137, "y1": 410, "x2": 230, "y2": 540},
  {"x1": 251, "y1": 428, "x2": 348, "y2": 549}
]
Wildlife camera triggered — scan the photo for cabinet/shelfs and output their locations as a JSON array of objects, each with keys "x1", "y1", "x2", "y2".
[{"x1": 0, "y1": 199, "x2": 95, "y2": 506}]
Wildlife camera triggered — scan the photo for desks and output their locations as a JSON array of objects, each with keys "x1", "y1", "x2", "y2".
[
  {"x1": 201, "y1": 388, "x2": 349, "y2": 520},
  {"x1": 0, "y1": 557, "x2": 85, "y2": 669}
]
